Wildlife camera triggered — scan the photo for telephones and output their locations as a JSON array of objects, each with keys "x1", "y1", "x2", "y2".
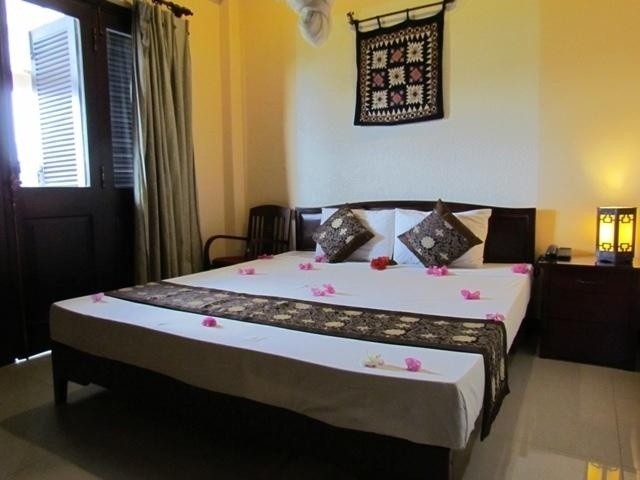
[{"x1": 544, "y1": 245, "x2": 571, "y2": 261}]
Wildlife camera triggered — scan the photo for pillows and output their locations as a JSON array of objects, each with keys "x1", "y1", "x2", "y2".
[
  {"x1": 315, "y1": 207, "x2": 394, "y2": 262},
  {"x1": 391, "y1": 208, "x2": 493, "y2": 267},
  {"x1": 396, "y1": 199, "x2": 483, "y2": 270},
  {"x1": 313, "y1": 203, "x2": 373, "y2": 263}
]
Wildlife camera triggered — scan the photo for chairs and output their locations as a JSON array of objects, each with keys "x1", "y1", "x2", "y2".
[{"x1": 202, "y1": 204, "x2": 293, "y2": 272}]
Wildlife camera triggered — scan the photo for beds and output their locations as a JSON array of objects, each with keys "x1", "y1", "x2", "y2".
[{"x1": 46, "y1": 199, "x2": 535, "y2": 479}]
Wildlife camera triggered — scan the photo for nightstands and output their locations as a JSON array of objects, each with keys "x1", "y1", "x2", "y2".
[{"x1": 537, "y1": 254, "x2": 640, "y2": 372}]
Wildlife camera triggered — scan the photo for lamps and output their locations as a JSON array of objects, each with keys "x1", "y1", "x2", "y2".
[{"x1": 595, "y1": 205, "x2": 637, "y2": 267}]
